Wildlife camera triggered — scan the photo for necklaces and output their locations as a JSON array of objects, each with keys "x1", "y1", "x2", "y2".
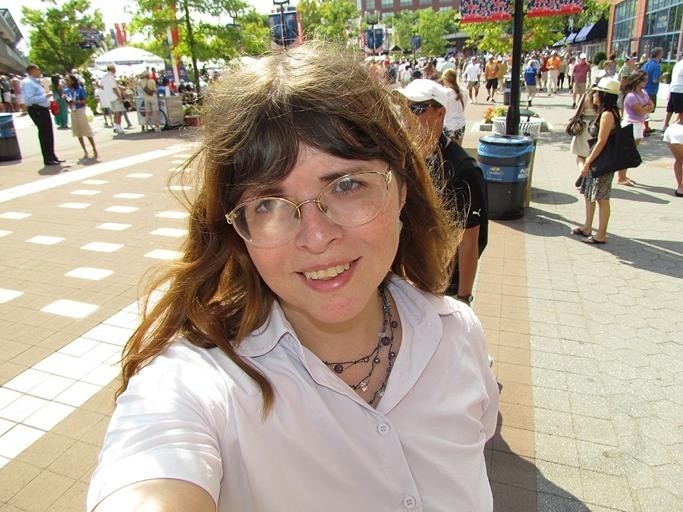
[{"x1": 320, "y1": 283, "x2": 399, "y2": 406}]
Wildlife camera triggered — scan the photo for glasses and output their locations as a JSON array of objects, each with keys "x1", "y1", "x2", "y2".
[
  {"x1": 408, "y1": 103, "x2": 440, "y2": 114},
  {"x1": 225, "y1": 168, "x2": 392, "y2": 248}
]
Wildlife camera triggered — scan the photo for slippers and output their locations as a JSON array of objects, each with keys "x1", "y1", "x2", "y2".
[
  {"x1": 570, "y1": 227, "x2": 587, "y2": 236},
  {"x1": 581, "y1": 236, "x2": 605, "y2": 243}
]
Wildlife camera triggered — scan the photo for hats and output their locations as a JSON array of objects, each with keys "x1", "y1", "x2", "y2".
[
  {"x1": 390, "y1": 78, "x2": 446, "y2": 112},
  {"x1": 591, "y1": 77, "x2": 622, "y2": 95},
  {"x1": 579, "y1": 53, "x2": 586, "y2": 59}
]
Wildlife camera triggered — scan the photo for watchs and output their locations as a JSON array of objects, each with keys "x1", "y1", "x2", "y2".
[{"x1": 456, "y1": 295, "x2": 474, "y2": 302}]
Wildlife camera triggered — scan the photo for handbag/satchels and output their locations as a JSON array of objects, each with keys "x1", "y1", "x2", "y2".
[
  {"x1": 586, "y1": 109, "x2": 642, "y2": 178},
  {"x1": 566, "y1": 94, "x2": 586, "y2": 135},
  {"x1": 51, "y1": 101, "x2": 60, "y2": 116},
  {"x1": 144, "y1": 79, "x2": 155, "y2": 96}
]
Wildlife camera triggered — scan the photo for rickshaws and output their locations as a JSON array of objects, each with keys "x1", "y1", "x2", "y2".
[{"x1": 134, "y1": 94, "x2": 184, "y2": 133}]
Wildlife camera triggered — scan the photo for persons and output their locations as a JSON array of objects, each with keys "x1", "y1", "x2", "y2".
[
  {"x1": 50, "y1": 73, "x2": 69, "y2": 129},
  {"x1": 2, "y1": 73, "x2": 54, "y2": 115},
  {"x1": 439, "y1": 68, "x2": 468, "y2": 145},
  {"x1": 519, "y1": 48, "x2": 591, "y2": 108},
  {"x1": 20, "y1": 64, "x2": 65, "y2": 165},
  {"x1": 663, "y1": 58, "x2": 683, "y2": 197},
  {"x1": 592, "y1": 47, "x2": 668, "y2": 136},
  {"x1": 84, "y1": 44, "x2": 500, "y2": 512},
  {"x1": 618, "y1": 69, "x2": 656, "y2": 186},
  {"x1": 571, "y1": 78, "x2": 603, "y2": 187},
  {"x1": 93, "y1": 65, "x2": 219, "y2": 135},
  {"x1": 62, "y1": 75, "x2": 98, "y2": 160},
  {"x1": 367, "y1": 53, "x2": 512, "y2": 104},
  {"x1": 386, "y1": 77, "x2": 490, "y2": 309},
  {"x1": 570, "y1": 77, "x2": 621, "y2": 244}
]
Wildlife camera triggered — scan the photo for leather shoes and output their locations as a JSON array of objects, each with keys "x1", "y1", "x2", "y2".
[
  {"x1": 55, "y1": 158, "x2": 65, "y2": 162},
  {"x1": 44, "y1": 160, "x2": 60, "y2": 165}
]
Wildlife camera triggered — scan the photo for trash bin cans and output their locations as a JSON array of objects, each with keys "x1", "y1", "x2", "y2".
[
  {"x1": 477, "y1": 133, "x2": 534, "y2": 220},
  {"x1": 490, "y1": 116, "x2": 542, "y2": 207},
  {"x1": 0, "y1": 113, "x2": 22, "y2": 163}
]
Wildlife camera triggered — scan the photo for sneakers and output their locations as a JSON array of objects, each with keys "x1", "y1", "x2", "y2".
[
  {"x1": 469, "y1": 86, "x2": 574, "y2": 105},
  {"x1": 111, "y1": 122, "x2": 161, "y2": 137}
]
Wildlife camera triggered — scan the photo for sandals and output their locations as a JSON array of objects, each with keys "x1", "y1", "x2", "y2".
[
  {"x1": 625, "y1": 177, "x2": 636, "y2": 184},
  {"x1": 618, "y1": 176, "x2": 634, "y2": 187}
]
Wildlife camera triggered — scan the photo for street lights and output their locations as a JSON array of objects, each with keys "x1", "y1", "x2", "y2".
[
  {"x1": 408, "y1": 17, "x2": 420, "y2": 63},
  {"x1": 365, "y1": 8, "x2": 379, "y2": 59},
  {"x1": 271, "y1": 0, "x2": 292, "y2": 50}
]
